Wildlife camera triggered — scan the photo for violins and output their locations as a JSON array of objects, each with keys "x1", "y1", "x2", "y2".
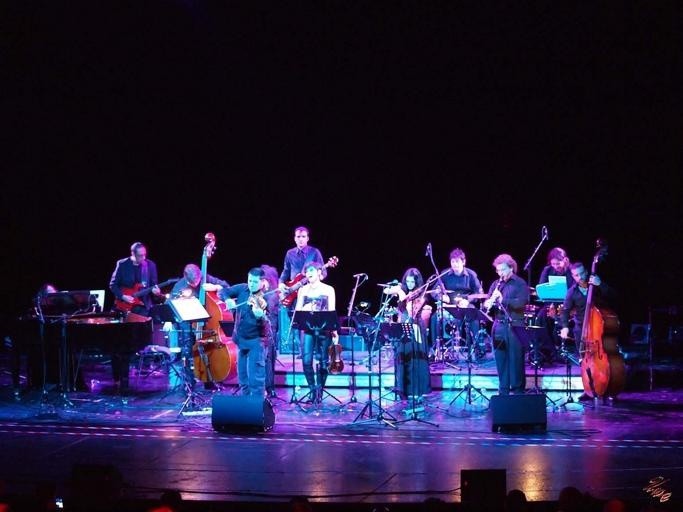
[
  {"x1": 327, "y1": 330, "x2": 344, "y2": 374},
  {"x1": 248, "y1": 292, "x2": 270, "y2": 324},
  {"x1": 408, "y1": 289, "x2": 454, "y2": 305}
]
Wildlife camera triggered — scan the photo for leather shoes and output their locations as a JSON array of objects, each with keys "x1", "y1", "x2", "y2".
[
  {"x1": 267, "y1": 390, "x2": 277, "y2": 398},
  {"x1": 607, "y1": 394, "x2": 617, "y2": 402},
  {"x1": 579, "y1": 394, "x2": 597, "y2": 401}
]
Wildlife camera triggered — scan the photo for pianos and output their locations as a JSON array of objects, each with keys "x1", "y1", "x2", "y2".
[{"x1": 10, "y1": 290, "x2": 153, "y2": 394}]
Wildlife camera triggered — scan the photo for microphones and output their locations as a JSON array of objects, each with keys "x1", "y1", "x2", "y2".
[
  {"x1": 544, "y1": 227, "x2": 550, "y2": 243},
  {"x1": 425, "y1": 243, "x2": 430, "y2": 256},
  {"x1": 352, "y1": 273, "x2": 364, "y2": 277}
]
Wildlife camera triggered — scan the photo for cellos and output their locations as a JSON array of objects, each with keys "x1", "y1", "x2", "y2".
[
  {"x1": 192, "y1": 232, "x2": 238, "y2": 384},
  {"x1": 578, "y1": 236, "x2": 626, "y2": 398}
]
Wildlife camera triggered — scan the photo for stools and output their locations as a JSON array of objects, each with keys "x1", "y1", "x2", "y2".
[{"x1": 0, "y1": 335, "x2": 40, "y2": 398}]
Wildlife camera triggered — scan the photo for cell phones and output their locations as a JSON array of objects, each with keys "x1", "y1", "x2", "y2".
[{"x1": 55, "y1": 496, "x2": 64, "y2": 508}]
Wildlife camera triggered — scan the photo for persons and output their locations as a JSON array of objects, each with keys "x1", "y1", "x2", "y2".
[
  {"x1": 287, "y1": 496, "x2": 313, "y2": 512},
  {"x1": 429, "y1": 250, "x2": 484, "y2": 360},
  {"x1": 382, "y1": 268, "x2": 432, "y2": 395},
  {"x1": 163, "y1": 264, "x2": 231, "y2": 391},
  {"x1": 484, "y1": 253, "x2": 529, "y2": 395},
  {"x1": 217, "y1": 267, "x2": 269, "y2": 397},
  {"x1": 294, "y1": 261, "x2": 339, "y2": 405},
  {"x1": 109, "y1": 242, "x2": 167, "y2": 370},
  {"x1": 506, "y1": 489, "x2": 626, "y2": 512},
  {"x1": 278, "y1": 225, "x2": 327, "y2": 359},
  {"x1": 0, "y1": 464, "x2": 183, "y2": 512},
  {"x1": 258, "y1": 264, "x2": 279, "y2": 396},
  {"x1": 560, "y1": 262, "x2": 615, "y2": 401},
  {"x1": 532, "y1": 248, "x2": 575, "y2": 327}
]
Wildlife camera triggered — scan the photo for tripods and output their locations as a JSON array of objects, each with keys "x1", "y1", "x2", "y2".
[
  {"x1": 295, "y1": 325, "x2": 342, "y2": 406},
  {"x1": 343, "y1": 333, "x2": 401, "y2": 431},
  {"x1": 446, "y1": 314, "x2": 490, "y2": 413},
  {"x1": 542, "y1": 349, "x2": 595, "y2": 413},
  {"x1": 516, "y1": 336, "x2": 559, "y2": 410},
  {"x1": 330, "y1": 327, "x2": 384, "y2": 414},
  {"x1": 230, "y1": 385, "x2": 288, "y2": 404},
  {"x1": 384, "y1": 293, "x2": 440, "y2": 428},
  {"x1": 154, "y1": 321, "x2": 212, "y2": 422},
  {"x1": 349, "y1": 334, "x2": 397, "y2": 423},
  {"x1": 268, "y1": 322, "x2": 318, "y2": 414}
]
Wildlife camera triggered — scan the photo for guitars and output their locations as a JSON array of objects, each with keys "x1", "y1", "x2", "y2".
[
  {"x1": 115, "y1": 274, "x2": 179, "y2": 313},
  {"x1": 280, "y1": 255, "x2": 340, "y2": 307}
]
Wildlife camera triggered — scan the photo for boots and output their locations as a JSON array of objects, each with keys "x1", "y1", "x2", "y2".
[
  {"x1": 314, "y1": 368, "x2": 328, "y2": 404},
  {"x1": 304, "y1": 369, "x2": 316, "y2": 402}
]
